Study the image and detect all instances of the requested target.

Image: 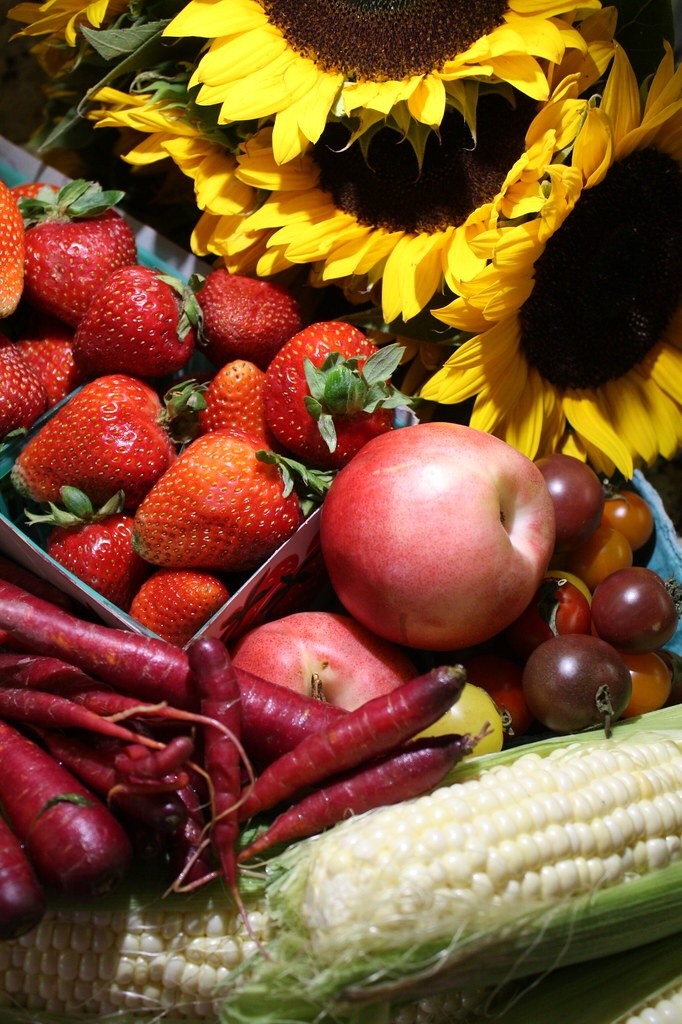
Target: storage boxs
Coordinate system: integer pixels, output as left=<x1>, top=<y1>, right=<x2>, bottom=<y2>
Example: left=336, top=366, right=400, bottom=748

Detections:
left=1, top=137, right=433, bottom=657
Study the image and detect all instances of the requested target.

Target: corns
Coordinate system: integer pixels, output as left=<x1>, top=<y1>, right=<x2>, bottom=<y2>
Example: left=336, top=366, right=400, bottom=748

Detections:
left=1, top=702, right=682, bottom=1024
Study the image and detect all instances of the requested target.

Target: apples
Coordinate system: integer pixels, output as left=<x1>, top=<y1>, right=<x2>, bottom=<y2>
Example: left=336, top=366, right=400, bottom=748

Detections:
left=230, top=419, right=553, bottom=714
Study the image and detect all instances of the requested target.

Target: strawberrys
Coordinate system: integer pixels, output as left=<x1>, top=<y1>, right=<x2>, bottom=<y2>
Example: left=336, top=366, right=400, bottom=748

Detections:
left=0, top=179, right=404, bottom=653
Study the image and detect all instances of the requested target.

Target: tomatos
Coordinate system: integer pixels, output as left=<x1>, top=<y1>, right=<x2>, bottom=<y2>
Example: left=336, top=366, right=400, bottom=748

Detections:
left=488, top=454, right=681, bottom=736
